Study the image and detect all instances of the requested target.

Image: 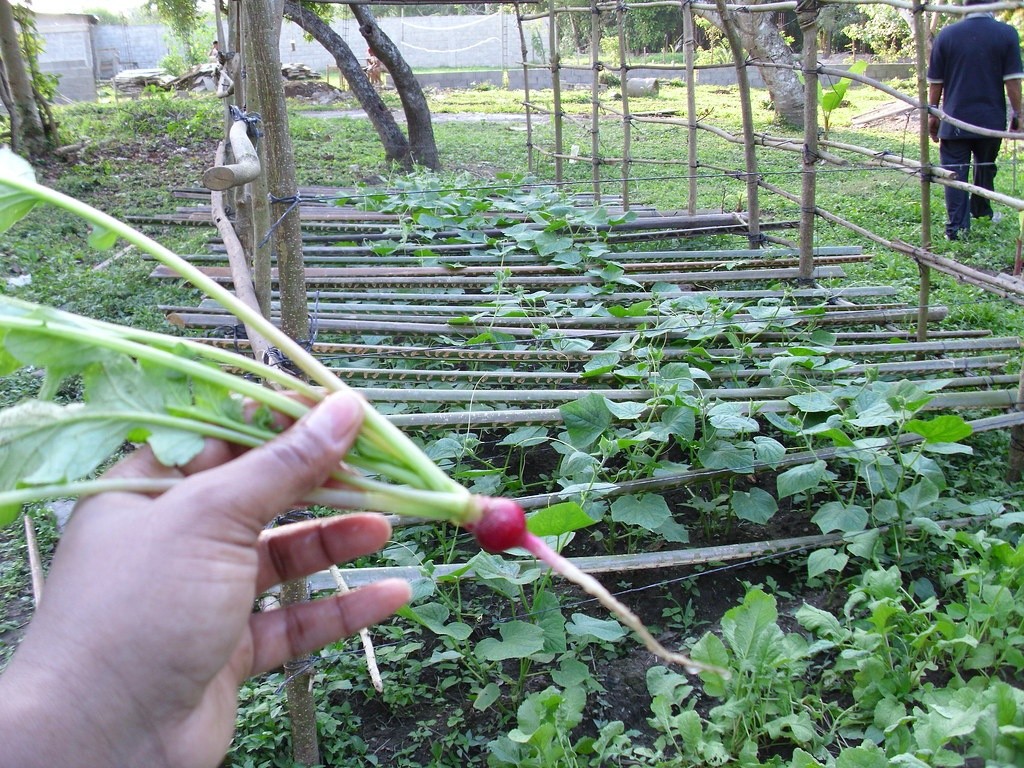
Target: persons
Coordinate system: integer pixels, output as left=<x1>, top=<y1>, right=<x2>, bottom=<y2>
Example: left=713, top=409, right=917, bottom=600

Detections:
left=366, top=48, right=383, bottom=82
left=923, top=0, right=1024, bottom=240
left=0, top=386, right=410, bottom=768
left=210, top=41, right=219, bottom=57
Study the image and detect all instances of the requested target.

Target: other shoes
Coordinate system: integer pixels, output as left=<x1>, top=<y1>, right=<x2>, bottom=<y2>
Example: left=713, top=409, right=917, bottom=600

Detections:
left=943, top=230, right=951, bottom=242
left=991, top=212, right=1005, bottom=222
